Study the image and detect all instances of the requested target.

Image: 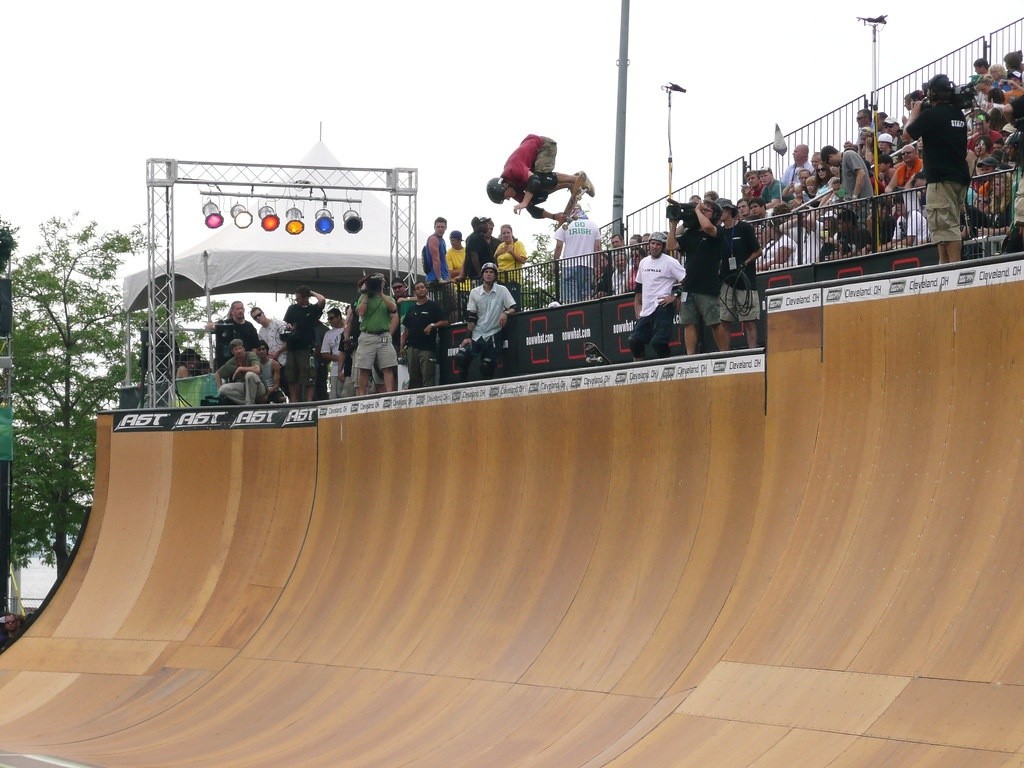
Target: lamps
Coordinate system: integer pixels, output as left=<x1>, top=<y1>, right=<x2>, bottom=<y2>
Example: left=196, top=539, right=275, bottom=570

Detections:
left=231, top=205, right=253, bottom=228
left=202, top=202, right=223, bottom=229
left=315, top=209, right=334, bottom=234
left=284, top=208, right=303, bottom=234
left=259, top=207, right=279, bottom=231
left=343, top=210, right=363, bottom=234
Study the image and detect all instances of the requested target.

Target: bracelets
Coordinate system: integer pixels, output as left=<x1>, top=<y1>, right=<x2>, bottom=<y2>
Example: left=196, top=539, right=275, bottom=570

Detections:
left=503, top=311, right=508, bottom=316
left=438, top=277, right=443, bottom=281
left=362, top=301, right=368, bottom=305
left=852, top=195, right=858, bottom=198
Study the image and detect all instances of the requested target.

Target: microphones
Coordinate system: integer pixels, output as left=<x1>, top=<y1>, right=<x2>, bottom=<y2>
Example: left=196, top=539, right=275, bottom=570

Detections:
left=667, top=198, right=678, bottom=204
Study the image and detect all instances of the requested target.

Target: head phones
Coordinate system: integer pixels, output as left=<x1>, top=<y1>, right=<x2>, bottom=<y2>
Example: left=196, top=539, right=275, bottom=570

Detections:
left=713, top=203, right=724, bottom=222
left=927, top=74, right=948, bottom=101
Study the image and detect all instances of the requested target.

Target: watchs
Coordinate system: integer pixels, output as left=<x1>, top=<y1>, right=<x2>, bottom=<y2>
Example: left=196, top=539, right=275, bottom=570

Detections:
left=673, top=292, right=678, bottom=298
left=430, top=323, right=435, bottom=327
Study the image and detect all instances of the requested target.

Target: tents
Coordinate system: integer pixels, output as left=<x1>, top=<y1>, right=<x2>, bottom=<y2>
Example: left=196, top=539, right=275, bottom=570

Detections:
left=121, top=142, right=431, bottom=388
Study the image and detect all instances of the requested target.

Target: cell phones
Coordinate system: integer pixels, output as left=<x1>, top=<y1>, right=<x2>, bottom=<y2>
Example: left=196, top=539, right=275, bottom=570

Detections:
left=1002, top=81, right=1007, bottom=85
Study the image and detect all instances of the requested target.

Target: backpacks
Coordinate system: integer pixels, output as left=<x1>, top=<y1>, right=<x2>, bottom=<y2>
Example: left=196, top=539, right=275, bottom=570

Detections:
left=421, top=235, right=441, bottom=276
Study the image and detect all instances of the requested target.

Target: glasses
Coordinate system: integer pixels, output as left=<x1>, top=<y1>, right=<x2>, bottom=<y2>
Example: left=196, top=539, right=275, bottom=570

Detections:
left=253, top=312, right=261, bottom=319
left=816, top=167, right=825, bottom=172
left=327, top=314, right=339, bottom=321
left=391, top=285, right=404, bottom=289
left=900, top=152, right=913, bottom=155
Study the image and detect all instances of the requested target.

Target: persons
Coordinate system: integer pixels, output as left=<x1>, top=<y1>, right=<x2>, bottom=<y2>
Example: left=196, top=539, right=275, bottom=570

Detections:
left=628, top=231, right=686, bottom=362
left=667, top=199, right=730, bottom=355
left=592, top=50, right=1024, bottom=299
left=554, top=203, right=603, bottom=305
left=903, top=74, right=970, bottom=265
left=451, top=261, right=517, bottom=380
left=175, top=215, right=527, bottom=405
left=714, top=198, right=762, bottom=350
left=487, top=135, right=595, bottom=223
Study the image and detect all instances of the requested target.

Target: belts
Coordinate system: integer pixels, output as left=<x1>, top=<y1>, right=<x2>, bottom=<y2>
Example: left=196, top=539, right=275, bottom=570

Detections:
left=363, top=330, right=389, bottom=335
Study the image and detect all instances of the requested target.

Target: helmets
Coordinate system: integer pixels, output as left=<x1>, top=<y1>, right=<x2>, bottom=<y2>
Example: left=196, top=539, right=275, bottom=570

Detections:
left=480, top=261, right=498, bottom=275
left=649, top=232, right=667, bottom=243
left=487, top=178, right=508, bottom=204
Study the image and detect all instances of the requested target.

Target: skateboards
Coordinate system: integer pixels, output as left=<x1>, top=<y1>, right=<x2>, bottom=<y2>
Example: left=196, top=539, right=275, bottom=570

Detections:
left=582, top=340, right=613, bottom=366
left=554, top=172, right=591, bottom=233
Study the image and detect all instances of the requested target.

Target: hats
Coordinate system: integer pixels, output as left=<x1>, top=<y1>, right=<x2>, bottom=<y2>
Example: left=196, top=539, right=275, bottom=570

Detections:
left=471, top=216, right=491, bottom=230
left=878, top=133, right=896, bottom=146
left=1007, top=70, right=1022, bottom=80
left=715, top=198, right=736, bottom=210
left=449, top=230, right=464, bottom=241
left=999, top=122, right=1016, bottom=133
left=976, top=157, right=999, bottom=168
left=859, top=126, right=872, bottom=135
left=228, top=338, right=244, bottom=349
left=820, top=211, right=837, bottom=218
left=754, top=166, right=773, bottom=174
